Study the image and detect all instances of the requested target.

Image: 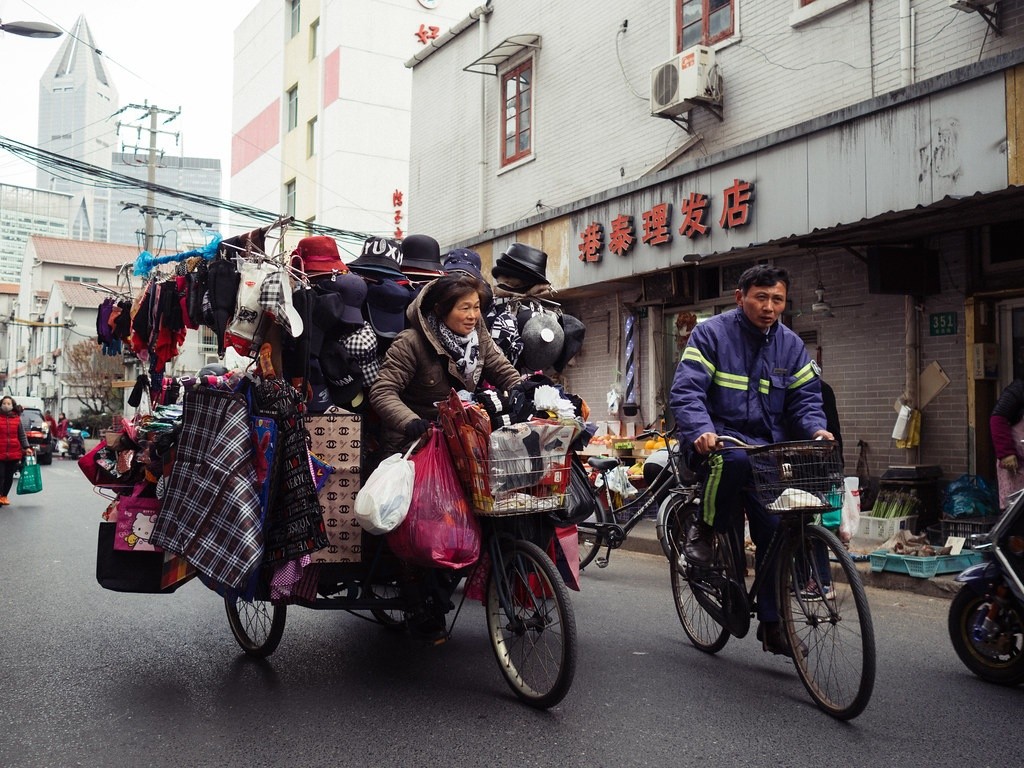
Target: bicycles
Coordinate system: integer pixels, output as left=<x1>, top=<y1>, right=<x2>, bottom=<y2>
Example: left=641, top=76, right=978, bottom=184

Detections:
left=670, top=436, right=879, bottom=721
left=573, top=424, right=724, bottom=582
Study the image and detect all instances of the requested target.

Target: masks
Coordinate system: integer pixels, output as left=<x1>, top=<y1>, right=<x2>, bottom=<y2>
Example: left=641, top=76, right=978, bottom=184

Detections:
left=1, top=404, right=13, bottom=412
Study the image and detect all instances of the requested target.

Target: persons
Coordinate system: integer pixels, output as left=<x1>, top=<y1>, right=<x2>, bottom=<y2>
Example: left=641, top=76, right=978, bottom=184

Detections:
left=670, top=265, right=834, bottom=658
left=990, top=346, right=1024, bottom=554
left=0, top=396, right=32, bottom=504
left=370, top=273, right=522, bottom=645
left=784, top=378, right=844, bottom=601
left=45, top=409, right=68, bottom=460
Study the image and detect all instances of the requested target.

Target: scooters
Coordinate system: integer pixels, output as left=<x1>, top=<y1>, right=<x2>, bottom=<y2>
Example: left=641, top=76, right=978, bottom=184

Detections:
left=66, top=421, right=89, bottom=460
left=945, top=488, right=1024, bottom=689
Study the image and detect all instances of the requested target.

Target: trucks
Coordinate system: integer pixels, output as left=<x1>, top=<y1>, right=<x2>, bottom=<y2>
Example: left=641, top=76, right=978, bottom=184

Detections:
left=11, top=395, right=46, bottom=415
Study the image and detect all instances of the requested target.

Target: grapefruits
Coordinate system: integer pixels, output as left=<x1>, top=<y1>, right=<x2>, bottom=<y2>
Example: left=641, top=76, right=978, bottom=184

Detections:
left=645, top=433, right=677, bottom=450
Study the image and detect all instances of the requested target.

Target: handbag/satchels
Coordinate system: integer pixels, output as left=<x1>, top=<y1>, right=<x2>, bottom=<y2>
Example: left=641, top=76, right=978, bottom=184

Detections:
left=386, top=428, right=481, bottom=570
left=821, top=482, right=842, bottom=528
left=489, top=420, right=575, bottom=495
left=837, top=476, right=861, bottom=542
left=17, top=455, right=43, bottom=495
left=353, top=437, right=421, bottom=535
left=113, top=478, right=165, bottom=553
left=547, top=451, right=596, bottom=526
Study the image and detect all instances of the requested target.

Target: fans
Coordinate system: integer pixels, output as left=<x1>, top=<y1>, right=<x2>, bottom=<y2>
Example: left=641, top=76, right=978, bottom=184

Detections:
left=784, top=250, right=866, bottom=319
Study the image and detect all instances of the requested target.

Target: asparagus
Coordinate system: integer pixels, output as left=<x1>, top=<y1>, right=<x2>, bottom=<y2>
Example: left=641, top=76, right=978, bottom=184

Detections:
left=627, top=461, right=644, bottom=475
left=870, top=488, right=921, bottom=519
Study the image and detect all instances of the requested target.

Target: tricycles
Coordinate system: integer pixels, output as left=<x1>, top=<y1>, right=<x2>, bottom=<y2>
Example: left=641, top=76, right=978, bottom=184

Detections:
left=176, top=380, right=580, bottom=711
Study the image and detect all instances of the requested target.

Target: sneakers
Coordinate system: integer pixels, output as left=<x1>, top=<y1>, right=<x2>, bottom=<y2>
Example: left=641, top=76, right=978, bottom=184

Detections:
left=787, top=578, right=813, bottom=596
left=799, top=580, right=836, bottom=602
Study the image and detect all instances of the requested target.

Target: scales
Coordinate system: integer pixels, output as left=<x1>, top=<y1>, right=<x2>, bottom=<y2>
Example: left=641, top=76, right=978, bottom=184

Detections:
left=879, top=464, right=944, bottom=480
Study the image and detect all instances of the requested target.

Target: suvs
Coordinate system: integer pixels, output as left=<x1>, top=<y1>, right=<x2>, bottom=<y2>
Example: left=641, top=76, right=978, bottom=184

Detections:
left=19, top=407, right=53, bottom=466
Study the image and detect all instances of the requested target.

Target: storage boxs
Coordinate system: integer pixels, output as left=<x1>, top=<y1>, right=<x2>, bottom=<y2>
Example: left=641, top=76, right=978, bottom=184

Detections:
left=855, top=511, right=918, bottom=541
left=871, top=550, right=986, bottom=580
left=938, top=511, right=998, bottom=546
left=613, top=498, right=632, bottom=524
left=632, top=489, right=659, bottom=518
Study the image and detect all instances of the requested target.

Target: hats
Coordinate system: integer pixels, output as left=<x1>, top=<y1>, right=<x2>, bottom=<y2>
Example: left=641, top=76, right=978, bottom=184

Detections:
left=258, top=232, right=586, bottom=412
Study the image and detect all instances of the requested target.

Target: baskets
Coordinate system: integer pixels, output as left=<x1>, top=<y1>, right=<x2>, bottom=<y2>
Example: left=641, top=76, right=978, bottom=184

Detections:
left=449, top=452, right=572, bottom=517
left=746, top=439, right=845, bottom=513
left=868, top=549, right=982, bottom=578
left=670, top=441, right=701, bottom=486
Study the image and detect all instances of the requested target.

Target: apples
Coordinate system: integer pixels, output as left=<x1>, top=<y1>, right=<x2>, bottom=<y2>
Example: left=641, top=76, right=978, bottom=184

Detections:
left=588, top=435, right=635, bottom=448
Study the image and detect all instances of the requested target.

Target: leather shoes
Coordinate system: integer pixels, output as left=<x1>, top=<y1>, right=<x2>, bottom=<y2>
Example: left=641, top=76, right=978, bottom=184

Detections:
left=683, top=519, right=713, bottom=567
left=0, top=496, right=9, bottom=505
left=756, top=621, right=808, bottom=658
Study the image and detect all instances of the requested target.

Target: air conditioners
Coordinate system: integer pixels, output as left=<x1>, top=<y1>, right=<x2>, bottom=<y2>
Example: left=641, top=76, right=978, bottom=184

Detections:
left=649, top=44, right=719, bottom=116
